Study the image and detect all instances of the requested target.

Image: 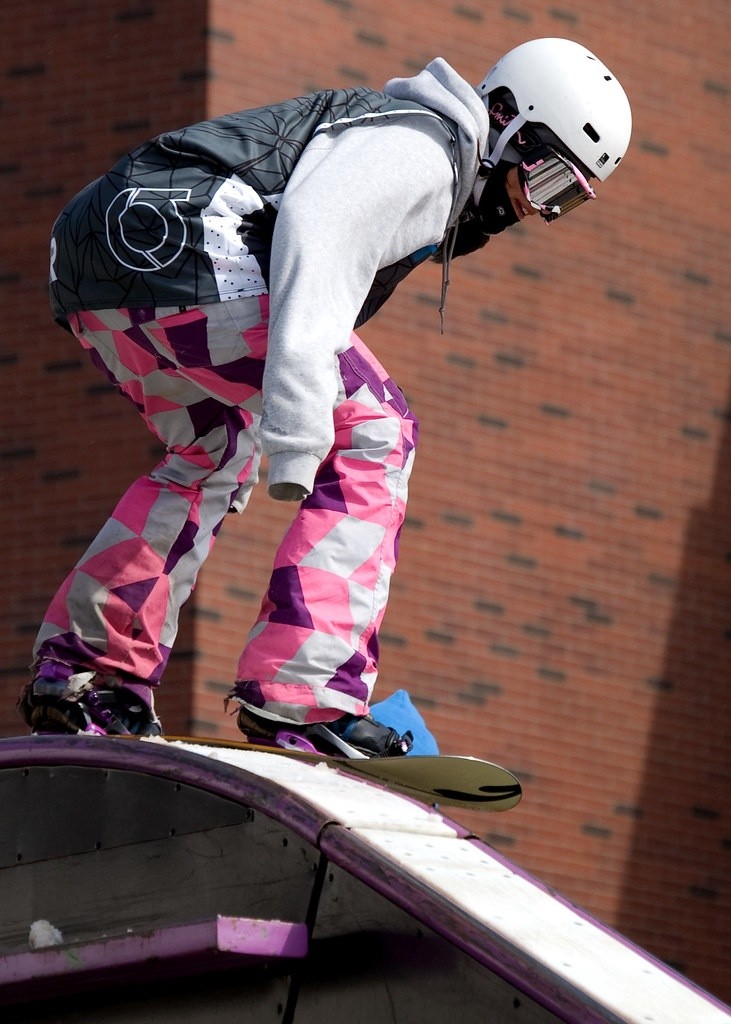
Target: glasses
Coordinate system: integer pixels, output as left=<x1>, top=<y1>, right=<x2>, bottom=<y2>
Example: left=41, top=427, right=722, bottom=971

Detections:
left=518, top=144, right=597, bottom=226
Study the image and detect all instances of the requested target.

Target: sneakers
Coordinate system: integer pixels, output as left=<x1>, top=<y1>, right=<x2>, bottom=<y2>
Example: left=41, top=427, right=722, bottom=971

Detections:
left=18, top=678, right=160, bottom=735
left=238, top=706, right=413, bottom=757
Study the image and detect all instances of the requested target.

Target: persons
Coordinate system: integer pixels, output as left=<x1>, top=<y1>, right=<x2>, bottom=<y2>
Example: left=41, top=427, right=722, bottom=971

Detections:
left=19, top=36, right=633, bottom=758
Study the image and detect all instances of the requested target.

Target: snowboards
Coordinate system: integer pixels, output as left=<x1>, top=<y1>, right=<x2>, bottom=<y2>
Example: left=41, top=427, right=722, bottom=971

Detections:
left=104, top=731, right=523, bottom=812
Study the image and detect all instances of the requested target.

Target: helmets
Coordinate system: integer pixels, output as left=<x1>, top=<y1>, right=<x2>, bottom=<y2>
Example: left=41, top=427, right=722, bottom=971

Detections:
left=477, top=37, right=633, bottom=182
